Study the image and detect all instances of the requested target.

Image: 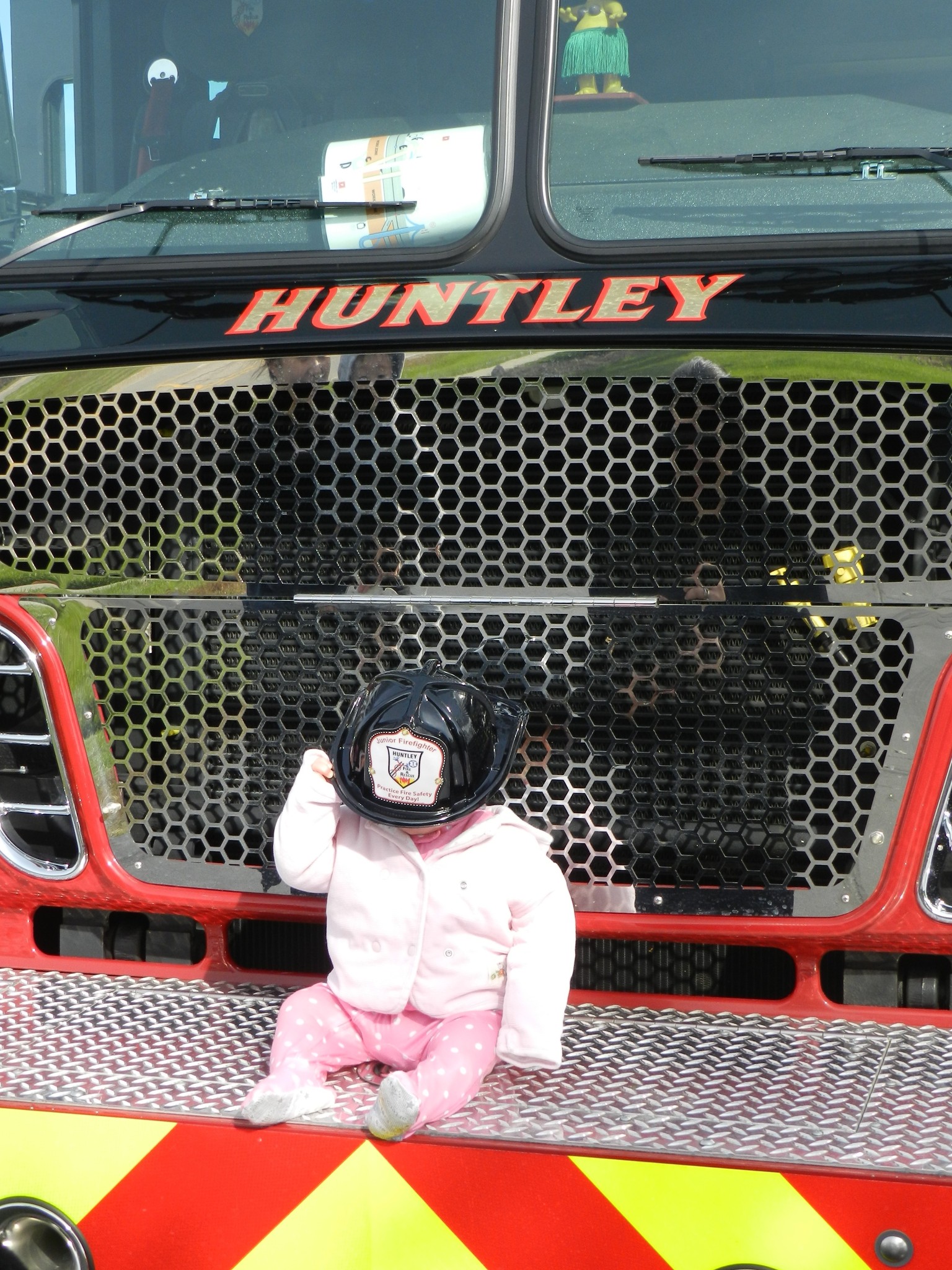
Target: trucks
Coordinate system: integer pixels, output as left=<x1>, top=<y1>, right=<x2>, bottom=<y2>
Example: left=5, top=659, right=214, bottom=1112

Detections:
left=0, top=1, right=952, bottom=1270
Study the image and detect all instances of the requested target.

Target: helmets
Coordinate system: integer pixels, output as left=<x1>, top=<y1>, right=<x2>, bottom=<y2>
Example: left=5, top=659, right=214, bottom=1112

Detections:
left=327, top=657, right=528, bottom=828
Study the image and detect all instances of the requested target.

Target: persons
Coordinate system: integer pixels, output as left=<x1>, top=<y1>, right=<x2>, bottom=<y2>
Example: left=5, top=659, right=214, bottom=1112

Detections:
left=239, top=659, right=576, bottom=1144
